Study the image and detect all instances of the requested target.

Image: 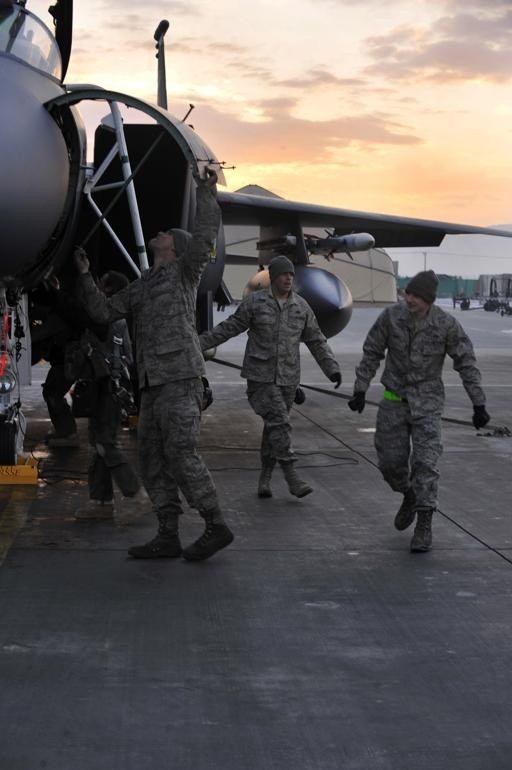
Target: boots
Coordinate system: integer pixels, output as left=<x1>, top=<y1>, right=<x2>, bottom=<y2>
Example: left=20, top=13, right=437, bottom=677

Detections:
left=258, top=468, right=272, bottom=497
left=411, top=506, right=433, bottom=551
left=280, top=460, right=313, bottom=498
left=395, top=487, right=416, bottom=531
left=183, top=507, right=233, bottom=560
left=128, top=520, right=181, bottom=558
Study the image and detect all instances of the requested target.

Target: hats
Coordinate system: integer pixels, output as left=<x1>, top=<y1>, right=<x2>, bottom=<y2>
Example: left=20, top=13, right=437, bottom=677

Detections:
left=405, top=271, right=440, bottom=305
left=171, top=228, right=192, bottom=259
left=268, top=256, right=295, bottom=280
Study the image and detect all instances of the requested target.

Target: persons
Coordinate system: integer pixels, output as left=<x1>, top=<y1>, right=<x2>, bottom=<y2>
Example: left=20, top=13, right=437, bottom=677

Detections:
left=30, top=265, right=83, bottom=449
left=70, top=271, right=140, bottom=521
left=197, top=255, right=344, bottom=497
left=71, top=164, right=233, bottom=564
left=347, top=269, right=492, bottom=553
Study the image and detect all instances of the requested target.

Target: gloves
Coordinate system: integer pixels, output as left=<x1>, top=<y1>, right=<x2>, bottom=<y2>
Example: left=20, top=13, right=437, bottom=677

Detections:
left=348, top=391, right=365, bottom=413
left=330, top=373, right=341, bottom=389
left=473, top=405, right=490, bottom=430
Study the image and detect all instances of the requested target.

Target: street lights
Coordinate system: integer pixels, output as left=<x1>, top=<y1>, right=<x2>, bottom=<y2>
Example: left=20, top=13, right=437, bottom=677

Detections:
left=421, top=251, right=428, bottom=273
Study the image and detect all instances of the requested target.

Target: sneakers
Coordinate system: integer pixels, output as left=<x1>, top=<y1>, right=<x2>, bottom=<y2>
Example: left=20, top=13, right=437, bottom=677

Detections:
left=49, top=433, right=78, bottom=447
left=75, top=500, right=116, bottom=518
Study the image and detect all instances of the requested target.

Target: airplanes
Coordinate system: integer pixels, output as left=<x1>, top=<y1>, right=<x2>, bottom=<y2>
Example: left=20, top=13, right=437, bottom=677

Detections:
left=0, top=0, right=512, bottom=464
